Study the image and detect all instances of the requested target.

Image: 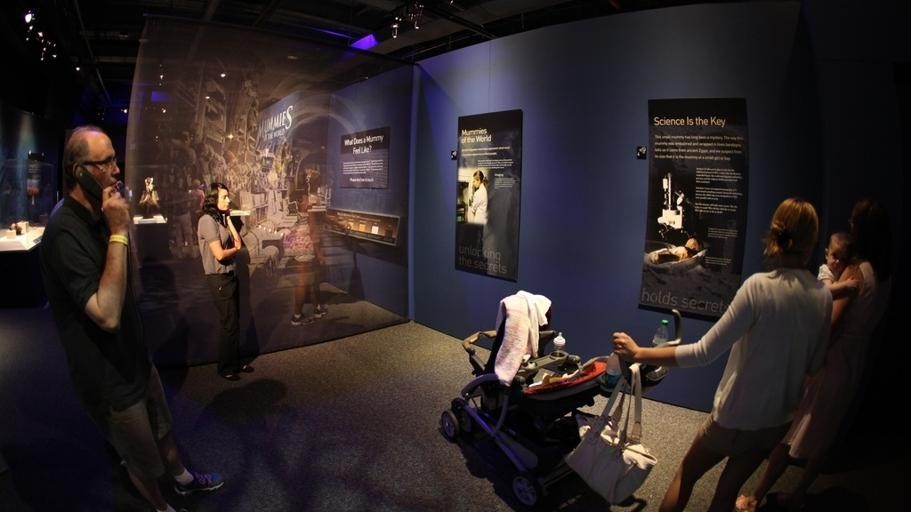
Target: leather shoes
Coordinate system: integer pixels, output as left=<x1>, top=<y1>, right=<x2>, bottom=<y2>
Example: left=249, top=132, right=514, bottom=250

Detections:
left=225, top=373, right=240, bottom=381
left=241, top=365, right=254, bottom=372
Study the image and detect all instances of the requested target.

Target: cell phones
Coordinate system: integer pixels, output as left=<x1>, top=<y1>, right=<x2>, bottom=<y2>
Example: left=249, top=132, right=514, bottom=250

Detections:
left=73, top=165, right=103, bottom=202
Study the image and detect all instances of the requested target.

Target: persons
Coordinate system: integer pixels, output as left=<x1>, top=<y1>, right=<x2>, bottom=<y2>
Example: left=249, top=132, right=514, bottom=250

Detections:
left=464, top=170, right=490, bottom=255
left=43, top=122, right=226, bottom=511
left=817, top=229, right=860, bottom=295
left=648, top=236, right=703, bottom=266
left=158, top=128, right=282, bottom=263
left=286, top=168, right=347, bottom=328
left=194, top=181, right=255, bottom=382
left=611, top=196, right=833, bottom=511
left=733, top=197, right=896, bottom=510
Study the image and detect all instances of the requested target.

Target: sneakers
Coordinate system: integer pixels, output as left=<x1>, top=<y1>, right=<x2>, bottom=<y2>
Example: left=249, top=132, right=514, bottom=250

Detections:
left=173, top=471, right=225, bottom=495
left=291, top=314, right=316, bottom=326
left=314, top=305, right=328, bottom=319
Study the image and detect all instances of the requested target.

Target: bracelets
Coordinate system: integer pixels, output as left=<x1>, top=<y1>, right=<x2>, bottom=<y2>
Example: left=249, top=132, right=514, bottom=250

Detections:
left=108, top=233, right=130, bottom=246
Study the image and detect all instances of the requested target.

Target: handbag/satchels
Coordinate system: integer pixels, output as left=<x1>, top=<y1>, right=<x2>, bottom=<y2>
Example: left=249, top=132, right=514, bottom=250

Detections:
left=565, top=414, right=659, bottom=505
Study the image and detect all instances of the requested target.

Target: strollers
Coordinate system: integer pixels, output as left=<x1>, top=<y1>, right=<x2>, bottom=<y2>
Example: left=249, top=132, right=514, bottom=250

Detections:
left=437, top=303, right=686, bottom=509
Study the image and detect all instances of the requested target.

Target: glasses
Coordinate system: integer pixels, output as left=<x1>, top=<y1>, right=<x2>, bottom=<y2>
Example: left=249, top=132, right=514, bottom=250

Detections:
left=82, top=157, right=117, bottom=170
